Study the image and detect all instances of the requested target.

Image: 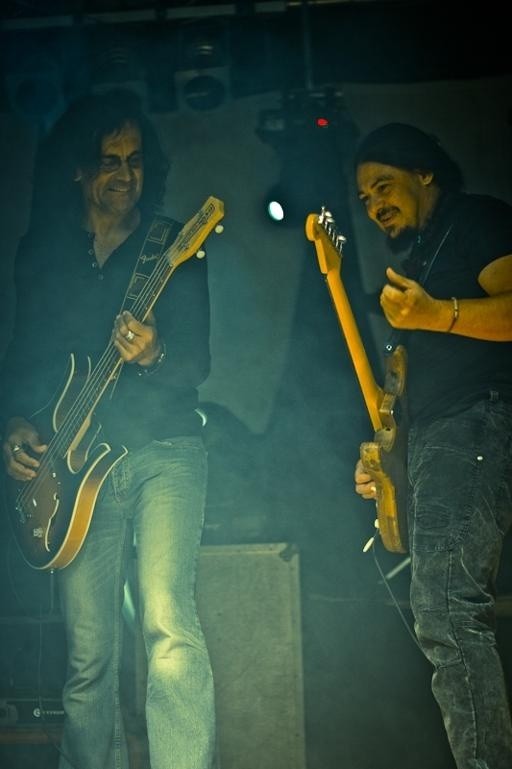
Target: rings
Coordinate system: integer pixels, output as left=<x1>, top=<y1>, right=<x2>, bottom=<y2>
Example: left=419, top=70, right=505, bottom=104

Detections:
left=12, top=444, right=25, bottom=457
left=125, top=330, right=135, bottom=341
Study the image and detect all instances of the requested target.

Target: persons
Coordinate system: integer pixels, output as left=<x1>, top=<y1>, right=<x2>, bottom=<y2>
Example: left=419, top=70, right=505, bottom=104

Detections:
left=353, top=123, right=512, bottom=769
left=0, top=95, right=218, bottom=769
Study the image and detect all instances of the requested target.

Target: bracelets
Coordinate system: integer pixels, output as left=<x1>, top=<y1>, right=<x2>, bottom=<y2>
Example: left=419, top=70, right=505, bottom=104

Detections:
left=135, top=343, right=165, bottom=377
left=444, top=297, right=460, bottom=334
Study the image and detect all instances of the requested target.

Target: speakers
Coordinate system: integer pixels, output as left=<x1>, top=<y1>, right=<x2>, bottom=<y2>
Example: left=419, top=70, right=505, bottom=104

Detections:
left=0, top=616, right=66, bottom=731
left=135, top=542, right=306, bottom=768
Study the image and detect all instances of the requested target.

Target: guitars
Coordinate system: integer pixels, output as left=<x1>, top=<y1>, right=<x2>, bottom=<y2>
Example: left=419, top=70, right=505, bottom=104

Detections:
left=1, top=196, right=225, bottom=570
left=306, top=206, right=409, bottom=554
